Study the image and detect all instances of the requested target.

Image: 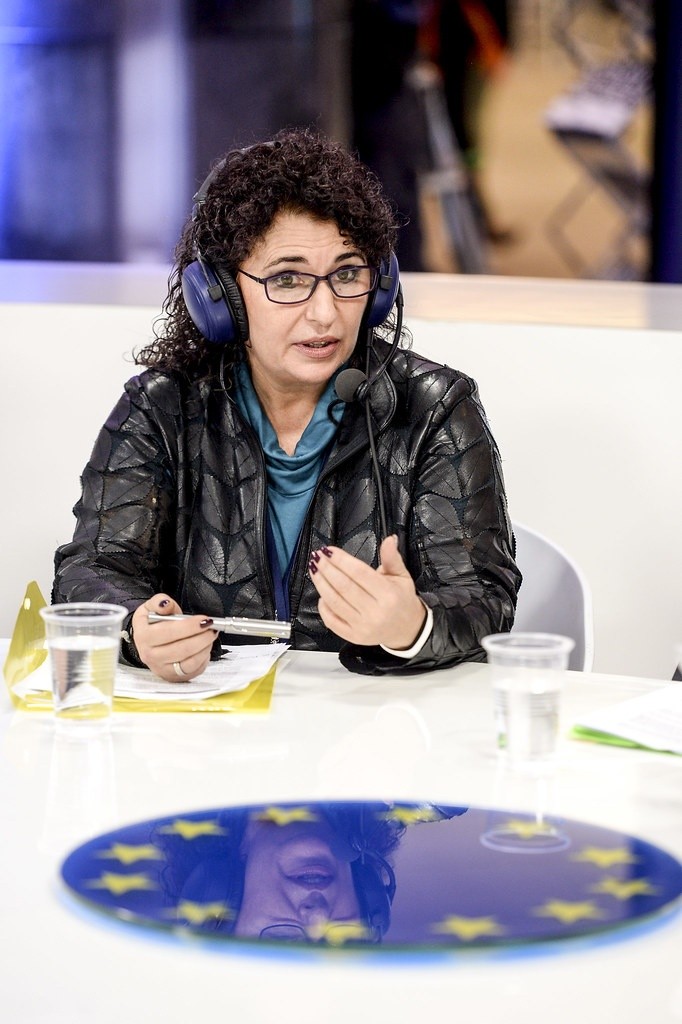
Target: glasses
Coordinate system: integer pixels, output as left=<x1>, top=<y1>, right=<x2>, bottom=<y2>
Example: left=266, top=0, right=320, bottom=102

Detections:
left=236, top=264, right=381, bottom=304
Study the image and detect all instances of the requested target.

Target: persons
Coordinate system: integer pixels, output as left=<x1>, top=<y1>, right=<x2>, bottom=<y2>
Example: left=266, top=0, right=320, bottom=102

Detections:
left=50, top=127, right=524, bottom=687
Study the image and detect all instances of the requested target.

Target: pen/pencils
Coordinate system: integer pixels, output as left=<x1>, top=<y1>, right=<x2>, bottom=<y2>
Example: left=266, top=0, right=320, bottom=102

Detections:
left=150, top=607, right=295, bottom=640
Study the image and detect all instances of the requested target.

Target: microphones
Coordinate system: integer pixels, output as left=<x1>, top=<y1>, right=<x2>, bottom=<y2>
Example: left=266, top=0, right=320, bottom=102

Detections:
left=335, top=285, right=404, bottom=401
left=328, top=830, right=397, bottom=901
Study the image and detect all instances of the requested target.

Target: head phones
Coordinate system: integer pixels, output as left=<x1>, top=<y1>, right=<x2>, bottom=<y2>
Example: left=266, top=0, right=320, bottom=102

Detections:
left=178, top=142, right=400, bottom=346
left=174, top=842, right=394, bottom=947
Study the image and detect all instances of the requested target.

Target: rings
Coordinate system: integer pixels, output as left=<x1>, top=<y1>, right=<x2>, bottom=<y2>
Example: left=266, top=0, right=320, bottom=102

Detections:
left=172, top=662, right=187, bottom=676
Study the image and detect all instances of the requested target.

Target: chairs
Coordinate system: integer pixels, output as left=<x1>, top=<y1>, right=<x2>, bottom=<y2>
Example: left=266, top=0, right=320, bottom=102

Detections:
left=505, top=524, right=597, bottom=670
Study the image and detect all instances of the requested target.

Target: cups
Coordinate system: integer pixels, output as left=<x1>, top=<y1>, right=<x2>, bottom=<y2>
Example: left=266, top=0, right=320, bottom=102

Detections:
left=40, top=604, right=128, bottom=724
left=481, top=632, right=574, bottom=758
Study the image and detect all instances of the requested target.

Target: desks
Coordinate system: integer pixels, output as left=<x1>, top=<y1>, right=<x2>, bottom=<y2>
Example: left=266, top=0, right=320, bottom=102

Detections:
left=0, top=635, right=681, bottom=1024
